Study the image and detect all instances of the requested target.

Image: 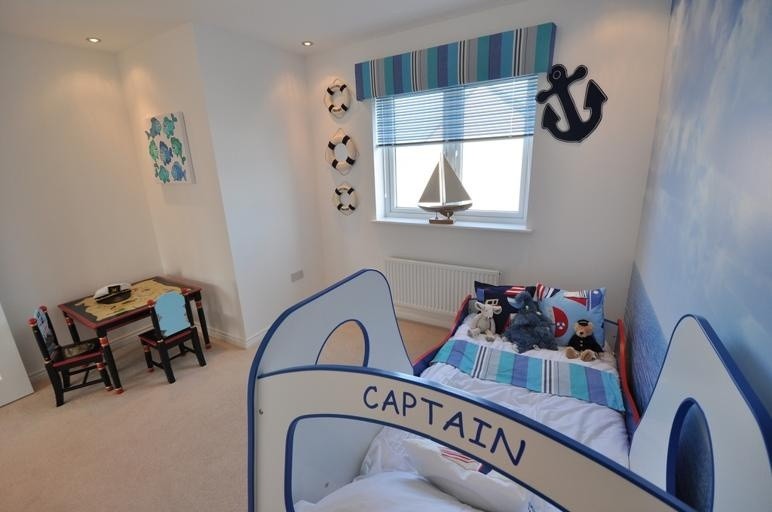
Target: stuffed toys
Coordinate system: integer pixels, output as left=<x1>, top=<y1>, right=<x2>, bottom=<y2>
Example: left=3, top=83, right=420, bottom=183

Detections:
left=566, top=319, right=603, bottom=361
left=502, top=290, right=560, bottom=352
left=468, top=301, right=502, bottom=341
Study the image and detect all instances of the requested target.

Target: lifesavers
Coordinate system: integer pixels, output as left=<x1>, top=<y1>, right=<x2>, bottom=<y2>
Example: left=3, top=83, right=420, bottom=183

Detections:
left=326, top=135, right=356, bottom=170
left=334, top=186, right=356, bottom=212
left=325, top=84, right=350, bottom=114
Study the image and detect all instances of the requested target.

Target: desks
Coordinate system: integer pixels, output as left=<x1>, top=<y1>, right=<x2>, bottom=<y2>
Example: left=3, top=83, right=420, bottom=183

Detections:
left=57, top=275, right=212, bottom=394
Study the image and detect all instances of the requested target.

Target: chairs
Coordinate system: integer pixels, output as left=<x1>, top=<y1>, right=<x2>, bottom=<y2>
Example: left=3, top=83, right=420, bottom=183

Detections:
left=136, top=288, right=206, bottom=384
left=27, top=305, right=113, bottom=407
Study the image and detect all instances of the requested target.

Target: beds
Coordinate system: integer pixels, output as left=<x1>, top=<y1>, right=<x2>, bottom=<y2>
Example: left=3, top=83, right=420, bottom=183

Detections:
left=247, top=268, right=772, bottom=512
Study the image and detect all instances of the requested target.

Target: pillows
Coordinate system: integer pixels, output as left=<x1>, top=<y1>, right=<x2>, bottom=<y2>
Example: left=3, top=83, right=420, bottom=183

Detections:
left=536, top=280, right=608, bottom=349
left=294, top=470, right=485, bottom=511
left=403, top=435, right=562, bottom=511
left=473, top=280, right=537, bottom=334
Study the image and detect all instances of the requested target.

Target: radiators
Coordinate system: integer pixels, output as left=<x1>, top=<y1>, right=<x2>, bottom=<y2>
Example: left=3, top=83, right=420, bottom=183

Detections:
left=383, top=257, right=501, bottom=317
